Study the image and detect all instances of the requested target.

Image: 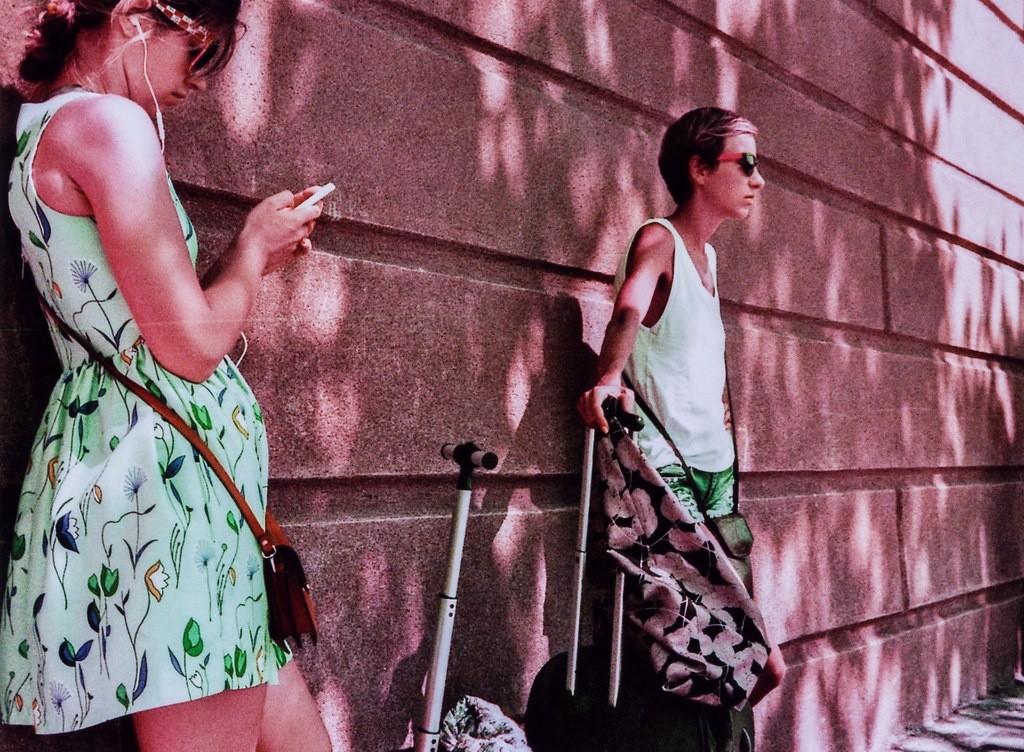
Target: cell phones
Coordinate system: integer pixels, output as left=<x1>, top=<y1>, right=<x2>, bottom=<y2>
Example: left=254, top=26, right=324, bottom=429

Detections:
left=293, top=183, right=336, bottom=212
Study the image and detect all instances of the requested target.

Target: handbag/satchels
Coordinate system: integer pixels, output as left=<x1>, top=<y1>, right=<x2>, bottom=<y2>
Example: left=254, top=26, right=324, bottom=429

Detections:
left=261, top=510, right=319, bottom=654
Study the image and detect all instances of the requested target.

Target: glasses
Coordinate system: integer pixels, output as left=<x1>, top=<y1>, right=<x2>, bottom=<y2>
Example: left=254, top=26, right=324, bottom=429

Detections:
left=150, top=0, right=220, bottom=76
left=716, top=150, right=759, bottom=177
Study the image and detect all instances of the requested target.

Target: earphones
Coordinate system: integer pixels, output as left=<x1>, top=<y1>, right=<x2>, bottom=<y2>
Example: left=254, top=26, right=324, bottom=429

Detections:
left=128, top=14, right=145, bottom=42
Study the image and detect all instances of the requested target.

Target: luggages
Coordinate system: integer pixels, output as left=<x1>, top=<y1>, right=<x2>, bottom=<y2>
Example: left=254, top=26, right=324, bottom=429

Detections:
left=525, top=401, right=758, bottom=752
left=418, top=437, right=530, bottom=752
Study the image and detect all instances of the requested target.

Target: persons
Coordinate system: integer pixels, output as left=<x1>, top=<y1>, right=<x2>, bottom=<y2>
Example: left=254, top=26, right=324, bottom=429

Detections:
left=579, top=106, right=786, bottom=705
left=0, top=0, right=334, bottom=752
